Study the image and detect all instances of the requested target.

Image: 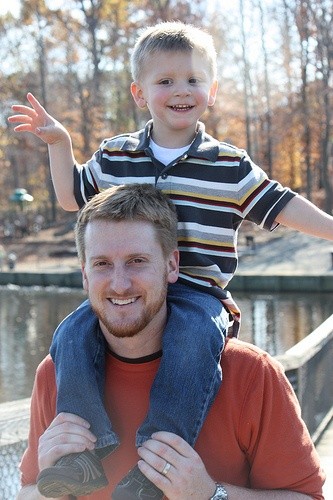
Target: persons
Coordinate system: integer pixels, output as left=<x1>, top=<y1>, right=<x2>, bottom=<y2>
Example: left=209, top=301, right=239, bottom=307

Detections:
left=17, top=185, right=326, bottom=500
left=7, top=22, right=332, bottom=500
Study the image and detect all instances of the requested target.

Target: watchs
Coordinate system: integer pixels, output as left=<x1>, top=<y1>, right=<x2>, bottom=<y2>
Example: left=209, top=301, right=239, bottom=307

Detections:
left=210, top=480, right=228, bottom=500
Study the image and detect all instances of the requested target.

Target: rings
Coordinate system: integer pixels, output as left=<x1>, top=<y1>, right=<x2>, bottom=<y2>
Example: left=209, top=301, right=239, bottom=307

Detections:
left=161, top=463, right=171, bottom=475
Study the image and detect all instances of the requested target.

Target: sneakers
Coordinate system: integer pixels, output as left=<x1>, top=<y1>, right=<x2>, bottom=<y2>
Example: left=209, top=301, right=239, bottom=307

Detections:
left=36, top=448, right=109, bottom=499
left=111, top=455, right=166, bottom=500
